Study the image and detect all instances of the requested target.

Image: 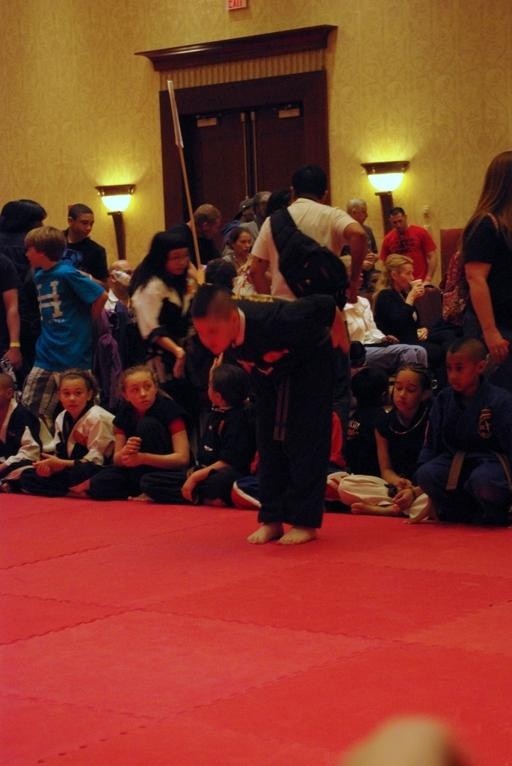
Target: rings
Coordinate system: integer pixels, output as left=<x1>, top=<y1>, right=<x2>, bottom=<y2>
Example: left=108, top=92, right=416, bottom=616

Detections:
left=494, top=350, right=499, bottom=353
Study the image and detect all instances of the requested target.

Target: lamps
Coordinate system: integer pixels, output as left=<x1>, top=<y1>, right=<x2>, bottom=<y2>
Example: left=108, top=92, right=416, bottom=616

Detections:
left=95, top=184, right=136, bottom=260
left=361, top=161, right=410, bottom=237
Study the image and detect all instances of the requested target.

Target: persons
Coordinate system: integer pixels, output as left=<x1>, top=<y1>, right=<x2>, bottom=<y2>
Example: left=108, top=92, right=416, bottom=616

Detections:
left=190, top=283, right=352, bottom=544
left=462, top=151, right=511, bottom=391
left=417, top=338, right=512, bottom=526
left=1, top=166, right=443, bottom=523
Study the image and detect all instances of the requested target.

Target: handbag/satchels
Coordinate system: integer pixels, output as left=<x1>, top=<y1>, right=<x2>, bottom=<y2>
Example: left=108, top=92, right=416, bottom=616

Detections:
left=442, top=212, right=499, bottom=326
left=277, top=232, right=347, bottom=311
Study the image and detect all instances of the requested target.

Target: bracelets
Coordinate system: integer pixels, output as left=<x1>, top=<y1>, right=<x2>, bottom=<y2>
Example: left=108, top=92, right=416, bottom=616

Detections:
left=410, top=488, right=417, bottom=498
left=9, top=343, right=20, bottom=347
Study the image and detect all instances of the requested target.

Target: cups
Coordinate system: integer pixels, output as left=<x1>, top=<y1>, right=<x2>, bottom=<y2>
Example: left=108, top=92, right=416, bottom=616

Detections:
left=408, top=278, right=423, bottom=296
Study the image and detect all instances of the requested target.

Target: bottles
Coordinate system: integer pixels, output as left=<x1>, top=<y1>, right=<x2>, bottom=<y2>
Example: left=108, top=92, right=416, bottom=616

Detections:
left=113, top=269, right=132, bottom=286
